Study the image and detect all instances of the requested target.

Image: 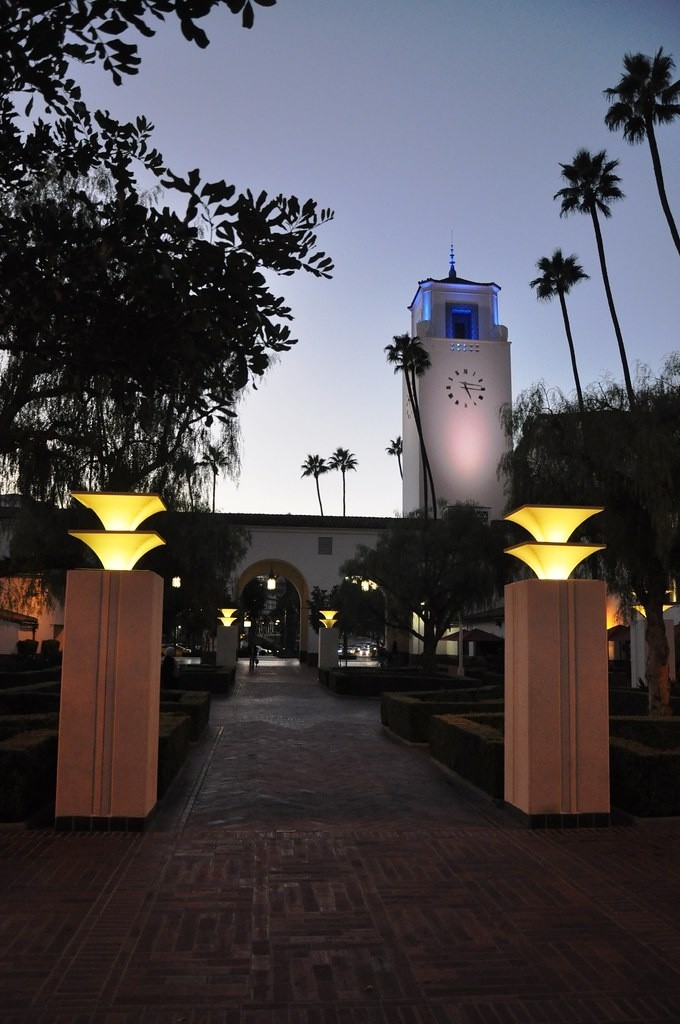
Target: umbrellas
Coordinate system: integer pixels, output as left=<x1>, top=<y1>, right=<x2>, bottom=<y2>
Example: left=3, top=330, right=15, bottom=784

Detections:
left=607, top=625, right=630, bottom=660
left=441, top=628, right=500, bottom=641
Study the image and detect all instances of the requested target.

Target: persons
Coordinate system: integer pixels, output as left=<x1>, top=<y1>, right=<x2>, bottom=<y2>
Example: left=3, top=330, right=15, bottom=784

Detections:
left=161, top=646, right=182, bottom=690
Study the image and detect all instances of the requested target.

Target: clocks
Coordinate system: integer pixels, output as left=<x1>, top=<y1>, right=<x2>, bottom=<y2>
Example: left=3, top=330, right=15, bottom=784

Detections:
left=446, top=369, right=486, bottom=407
